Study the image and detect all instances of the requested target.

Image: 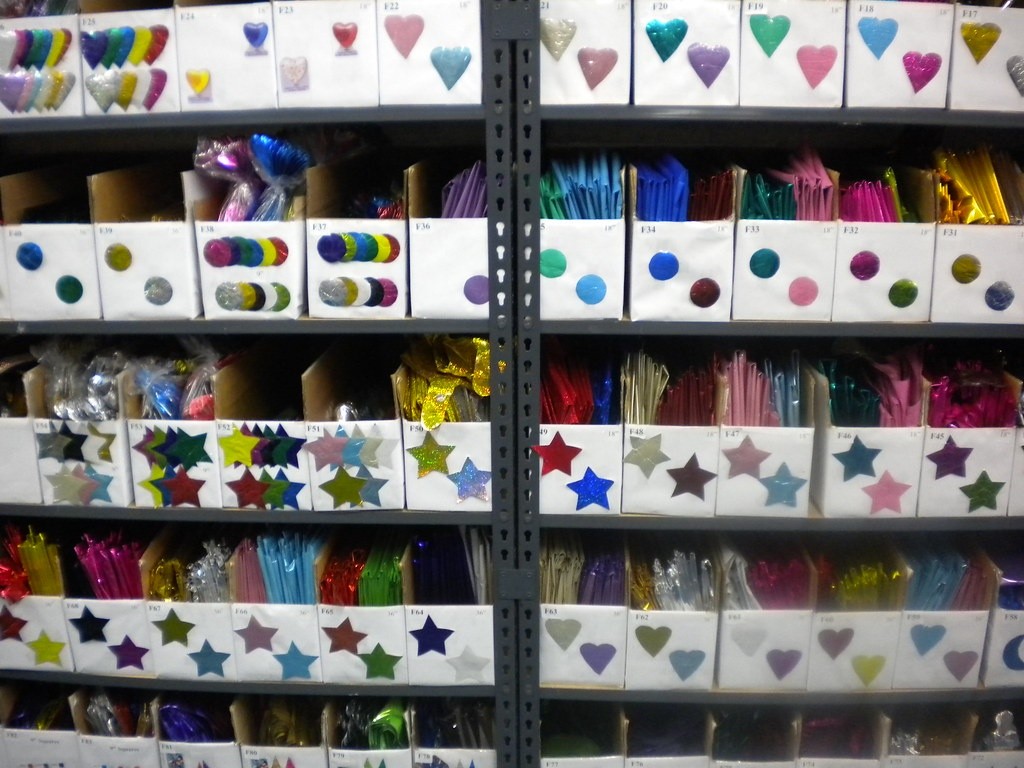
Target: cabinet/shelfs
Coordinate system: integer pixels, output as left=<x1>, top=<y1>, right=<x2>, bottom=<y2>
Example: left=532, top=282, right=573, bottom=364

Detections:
left=0, top=0, right=512, bottom=768
left=510, top=0, right=1024, bottom=768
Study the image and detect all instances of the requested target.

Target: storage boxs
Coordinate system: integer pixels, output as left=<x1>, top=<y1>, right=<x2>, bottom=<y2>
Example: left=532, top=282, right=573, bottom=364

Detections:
left=0, top=0, right=497, bottom=768
left=539, top=0, right=1024, bottom=768
